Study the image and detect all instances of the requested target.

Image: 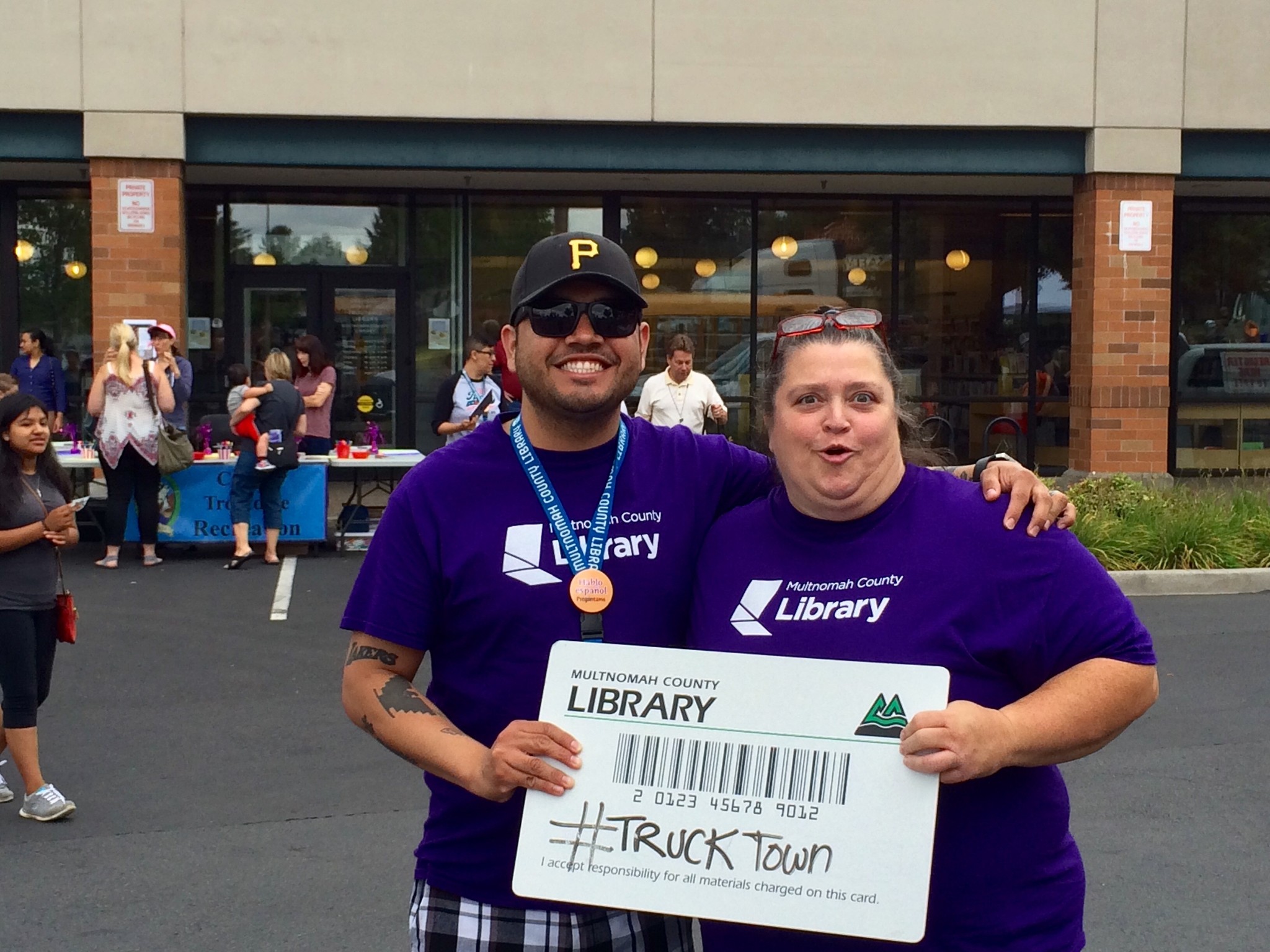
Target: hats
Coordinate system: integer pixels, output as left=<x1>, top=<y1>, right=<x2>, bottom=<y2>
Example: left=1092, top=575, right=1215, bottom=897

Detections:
left=510, top=230, right=649, bottom=325
left=146, top=324, right=176, bottom=339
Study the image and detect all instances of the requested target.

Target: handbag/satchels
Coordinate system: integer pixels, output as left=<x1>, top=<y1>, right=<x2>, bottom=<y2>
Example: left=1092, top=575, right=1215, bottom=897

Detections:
left=157, top=424, right=193, bottom=474
left=55, top=592, right=77, bottom=644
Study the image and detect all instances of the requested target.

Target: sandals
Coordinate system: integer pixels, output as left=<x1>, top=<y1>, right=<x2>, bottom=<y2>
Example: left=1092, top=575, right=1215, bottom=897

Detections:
left=96, top=556, right=118, bottom=569
left=143, top=554, right=163, bottom=567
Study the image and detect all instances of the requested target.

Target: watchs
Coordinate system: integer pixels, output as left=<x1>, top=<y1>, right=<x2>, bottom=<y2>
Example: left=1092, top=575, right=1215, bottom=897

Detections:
left=972, top=453, right=1022, bottom=483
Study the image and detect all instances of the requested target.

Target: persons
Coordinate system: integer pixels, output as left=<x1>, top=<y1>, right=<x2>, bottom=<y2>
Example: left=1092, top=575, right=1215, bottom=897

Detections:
left=340, top=231, right=1076, bottom=952
left=686, top=305, right=1159, bottom=952
left=0, top=392, right=80, bottom=819
left=87, top=323, right=176, bottom=568
left=1024, top=329, right=1069, bottom=447
left=292, top=334, right=337, bottom=455
left=147, top=323, right=193, bottom=428
left=224, top=351, right=307, bottom=570
left=430, top=334, right=504, bottom=445
left=226, top=364, right=276, bottom=470
left=0, top=373, right=19, bottom=399
left=491, top=340, right=523, bottom=412
left=635, top=334, right=728, bottom=436
left=9, top=328, right=67, bottom=441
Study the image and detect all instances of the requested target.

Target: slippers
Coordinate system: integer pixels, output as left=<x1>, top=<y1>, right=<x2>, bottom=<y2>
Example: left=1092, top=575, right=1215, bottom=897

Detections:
left=261, top=556, right=281, bottom=565
left=224, top=550, right=255, bottom=569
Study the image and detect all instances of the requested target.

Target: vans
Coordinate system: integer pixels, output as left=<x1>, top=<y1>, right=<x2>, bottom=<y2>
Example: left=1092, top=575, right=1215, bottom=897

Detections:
left=1175, top=331, right=1270, bottom=402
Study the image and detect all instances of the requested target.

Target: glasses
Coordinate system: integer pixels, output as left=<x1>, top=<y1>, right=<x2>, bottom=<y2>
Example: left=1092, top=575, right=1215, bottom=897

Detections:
left=474, top=349, right=494, bottom=357
left=514, top=293, right=640, bottom=337
left=772, top=308, right=886, bottom=361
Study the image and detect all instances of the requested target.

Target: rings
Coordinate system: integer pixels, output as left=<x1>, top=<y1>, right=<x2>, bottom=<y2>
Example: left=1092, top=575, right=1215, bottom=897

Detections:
left=1049, top=490, right=1059, bottom=496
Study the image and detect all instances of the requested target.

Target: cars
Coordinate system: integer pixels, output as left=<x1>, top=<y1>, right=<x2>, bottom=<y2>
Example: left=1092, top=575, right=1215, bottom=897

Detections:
left=628, top=331, right=779, bottom=403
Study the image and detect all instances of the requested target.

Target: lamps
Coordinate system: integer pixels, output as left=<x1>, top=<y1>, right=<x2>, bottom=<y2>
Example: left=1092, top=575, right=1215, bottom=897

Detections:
left=771, top=216, right=799, bottom=260
left=642, top=273, right=660, bottom=290
left=253, top=253, right=276, bottom=265
left=945, top=235, right=970, bottom=271
left=635, top=245, right=658, bottom=268
left=345, top=243, right=368, bottom=265
left=17, top=239, right=35, bottom=261
left=849, top=260, right=866, bottom=285
left=696, top=258, right=717, bottom=277
left=65, top=260, right=87, bottom=279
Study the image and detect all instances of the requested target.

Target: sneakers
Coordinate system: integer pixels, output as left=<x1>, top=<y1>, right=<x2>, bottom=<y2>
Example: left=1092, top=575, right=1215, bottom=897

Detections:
left=254, top=459, right=276, bottom=471
left=18, top=782, right=76, bottom=821
left=0, top=760, right=13, bottom=803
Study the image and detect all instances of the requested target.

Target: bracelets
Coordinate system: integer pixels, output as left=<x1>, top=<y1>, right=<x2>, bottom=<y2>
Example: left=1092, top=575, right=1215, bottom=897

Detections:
left=41, top=520, right=50, bottom=531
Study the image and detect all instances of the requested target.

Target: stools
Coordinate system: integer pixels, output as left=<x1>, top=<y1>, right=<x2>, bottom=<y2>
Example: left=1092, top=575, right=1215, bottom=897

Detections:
left=989, top=412, right=1028, bottom=462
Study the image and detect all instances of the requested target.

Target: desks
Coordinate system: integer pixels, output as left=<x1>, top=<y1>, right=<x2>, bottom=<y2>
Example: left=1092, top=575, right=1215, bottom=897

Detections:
left=50, top=448, right=426, bottom=555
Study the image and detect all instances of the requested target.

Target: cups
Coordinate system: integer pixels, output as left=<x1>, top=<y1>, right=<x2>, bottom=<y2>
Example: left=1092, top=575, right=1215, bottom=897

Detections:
left=218, top=449, right=231, bottom=460
left=81, top=444, right=95, bottom=459
left=336, top=445, right=350, bottom=458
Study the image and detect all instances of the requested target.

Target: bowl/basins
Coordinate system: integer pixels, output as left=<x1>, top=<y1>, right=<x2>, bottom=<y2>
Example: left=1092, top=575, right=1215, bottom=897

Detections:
left=351, top=452, right=370, bottom=459
left=194, top=452, right=206, bottom=460
